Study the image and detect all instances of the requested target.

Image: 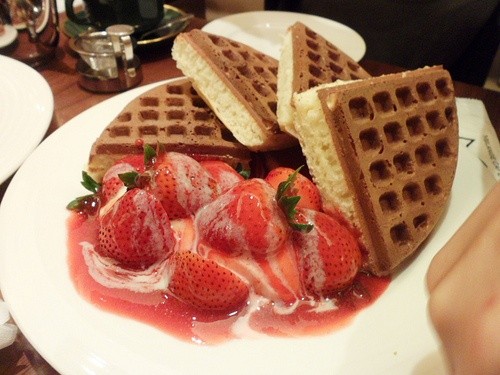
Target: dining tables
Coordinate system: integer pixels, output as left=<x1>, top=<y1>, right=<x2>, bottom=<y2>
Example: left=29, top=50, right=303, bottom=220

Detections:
left=0, top=0, right=500, bottom=375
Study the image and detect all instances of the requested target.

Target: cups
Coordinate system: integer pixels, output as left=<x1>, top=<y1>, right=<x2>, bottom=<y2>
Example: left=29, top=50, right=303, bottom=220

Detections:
left=68, top=22, right=143, bottom=92
left=65, top=0, right=164, bottom=34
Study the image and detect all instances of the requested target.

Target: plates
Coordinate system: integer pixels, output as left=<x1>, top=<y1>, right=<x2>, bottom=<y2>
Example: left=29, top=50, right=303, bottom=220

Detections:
left=0, top=53, right=54, bottom=185
left=202, top=9, right=367, bottom=67
left=0, top=77, right=461, bottom=375
left=61, top=4, right=192, bottom=54
left=0, top=23, right=17, bottom=48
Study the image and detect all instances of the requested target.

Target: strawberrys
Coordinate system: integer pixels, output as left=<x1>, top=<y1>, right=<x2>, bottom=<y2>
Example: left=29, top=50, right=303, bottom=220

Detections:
left=67, top=146, right=361, bottom=311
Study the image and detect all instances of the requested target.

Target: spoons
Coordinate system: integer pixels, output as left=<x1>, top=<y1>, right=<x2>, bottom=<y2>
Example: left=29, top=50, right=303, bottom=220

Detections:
left=131, top=15, right=194, bottom=40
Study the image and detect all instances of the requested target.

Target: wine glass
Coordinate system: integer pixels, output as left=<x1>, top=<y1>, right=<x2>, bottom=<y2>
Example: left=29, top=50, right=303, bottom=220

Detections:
left=0, top=0, right=59, bottom=67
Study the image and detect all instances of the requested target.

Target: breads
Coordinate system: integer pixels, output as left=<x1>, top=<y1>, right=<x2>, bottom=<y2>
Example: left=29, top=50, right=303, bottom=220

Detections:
left=171, top=28, right=299, bottom=153
left=276, top=21, right=375, bottom=141
left=85, top=79, right=253, bottom=180
left=294, top=64, right=458, bottom=278
